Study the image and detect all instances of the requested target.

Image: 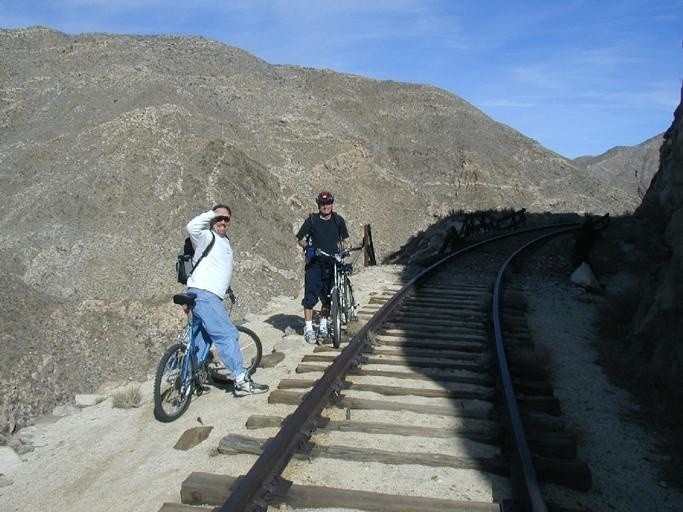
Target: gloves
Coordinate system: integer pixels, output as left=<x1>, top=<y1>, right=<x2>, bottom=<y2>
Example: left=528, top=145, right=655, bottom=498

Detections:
left=304, top=245, right=315, bottom=261
left=343, top=263, right=353, bottom=274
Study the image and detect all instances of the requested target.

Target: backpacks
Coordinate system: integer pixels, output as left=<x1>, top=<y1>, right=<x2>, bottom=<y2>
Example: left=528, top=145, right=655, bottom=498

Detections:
left=176, top=231, right=216, bottom=285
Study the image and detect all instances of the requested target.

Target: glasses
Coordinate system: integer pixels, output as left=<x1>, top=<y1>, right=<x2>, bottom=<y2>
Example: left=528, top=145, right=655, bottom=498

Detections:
left=215, top=217, right=230, bottom=222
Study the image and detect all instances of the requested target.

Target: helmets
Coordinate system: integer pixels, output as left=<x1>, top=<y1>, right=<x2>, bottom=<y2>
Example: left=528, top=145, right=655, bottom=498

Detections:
left=315, top=192, right=334, bottom=205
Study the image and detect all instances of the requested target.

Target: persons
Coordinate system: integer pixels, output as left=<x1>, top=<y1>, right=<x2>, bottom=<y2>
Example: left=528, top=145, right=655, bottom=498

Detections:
left=296, top=191, right=350, bottom=345
left=178, top=204, right=272, bottom=397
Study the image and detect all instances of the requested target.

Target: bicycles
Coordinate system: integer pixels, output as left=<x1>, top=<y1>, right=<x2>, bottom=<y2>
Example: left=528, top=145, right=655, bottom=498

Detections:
left=315, top=247, right=362, bottom=348
left=154, top=285, right=262, bottom=422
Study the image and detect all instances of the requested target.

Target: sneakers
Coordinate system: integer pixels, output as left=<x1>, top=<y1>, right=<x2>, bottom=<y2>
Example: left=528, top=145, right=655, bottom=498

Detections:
left=304, top=326, right=328, bottom=344
left=199, top=386, right=211, bottom=394
left=235, top=373, right=269, bottom=397
